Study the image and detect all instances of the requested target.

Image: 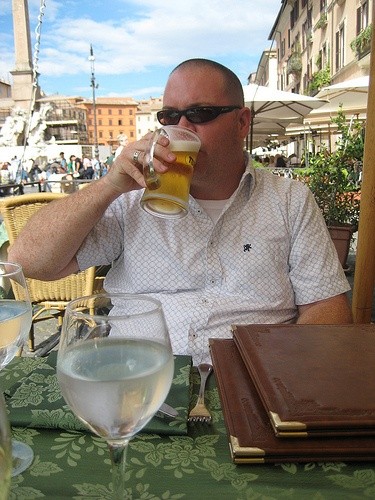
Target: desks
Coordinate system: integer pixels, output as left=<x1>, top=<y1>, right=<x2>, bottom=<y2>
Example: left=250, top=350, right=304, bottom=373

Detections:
left=0, top=357, right=374, bottom=500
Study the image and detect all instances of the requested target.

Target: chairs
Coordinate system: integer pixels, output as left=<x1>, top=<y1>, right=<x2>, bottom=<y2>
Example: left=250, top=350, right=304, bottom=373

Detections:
left=1, top=192, right=111, bottom=352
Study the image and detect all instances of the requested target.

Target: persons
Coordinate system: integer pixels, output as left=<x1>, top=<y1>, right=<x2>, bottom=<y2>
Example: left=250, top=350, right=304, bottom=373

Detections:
left=6, top=58, right=355, bottom=367
left=253, top=153, right=301, bottom=168
left=0, top=150, right=117, bottom=185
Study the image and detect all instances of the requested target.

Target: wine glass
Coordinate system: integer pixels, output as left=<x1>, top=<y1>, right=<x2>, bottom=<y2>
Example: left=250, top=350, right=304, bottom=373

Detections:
left=56, top=293, right=175, bottom=500
left=0, top=260, right=34, bottom=476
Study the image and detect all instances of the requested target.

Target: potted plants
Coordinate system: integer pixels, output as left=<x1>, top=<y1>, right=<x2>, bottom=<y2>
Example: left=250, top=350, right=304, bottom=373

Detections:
left=294, top=100, right=366, bottom=268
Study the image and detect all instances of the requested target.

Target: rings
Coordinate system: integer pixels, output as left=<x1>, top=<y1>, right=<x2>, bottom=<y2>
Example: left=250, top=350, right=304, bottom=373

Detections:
left=133, top=150, right=142, bottom=161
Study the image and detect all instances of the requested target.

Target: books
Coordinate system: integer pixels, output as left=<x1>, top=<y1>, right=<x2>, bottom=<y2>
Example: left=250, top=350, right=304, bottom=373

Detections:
left=207, top=322, right=375, bottom=464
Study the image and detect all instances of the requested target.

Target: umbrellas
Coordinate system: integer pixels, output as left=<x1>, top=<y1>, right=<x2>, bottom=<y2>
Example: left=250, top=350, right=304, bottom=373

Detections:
left=241, top=75, right=370, bottom=155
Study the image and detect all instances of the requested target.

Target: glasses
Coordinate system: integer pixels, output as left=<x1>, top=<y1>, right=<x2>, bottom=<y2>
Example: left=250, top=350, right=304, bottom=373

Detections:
left=157, top=105, right=245, bottom=127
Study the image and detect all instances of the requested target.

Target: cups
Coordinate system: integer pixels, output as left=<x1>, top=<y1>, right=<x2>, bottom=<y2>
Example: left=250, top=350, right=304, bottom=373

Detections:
left=0, top=390, right=13, bottom=500
left=139, top=124, right=203, bottom=220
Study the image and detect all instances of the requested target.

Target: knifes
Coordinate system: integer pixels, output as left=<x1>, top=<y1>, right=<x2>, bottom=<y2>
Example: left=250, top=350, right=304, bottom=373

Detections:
left=158, top=402, right=178, bottom=418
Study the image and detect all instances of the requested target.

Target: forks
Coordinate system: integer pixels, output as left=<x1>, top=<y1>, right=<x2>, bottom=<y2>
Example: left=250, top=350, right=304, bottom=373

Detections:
left=186, top=362, right=213, bottom=424
